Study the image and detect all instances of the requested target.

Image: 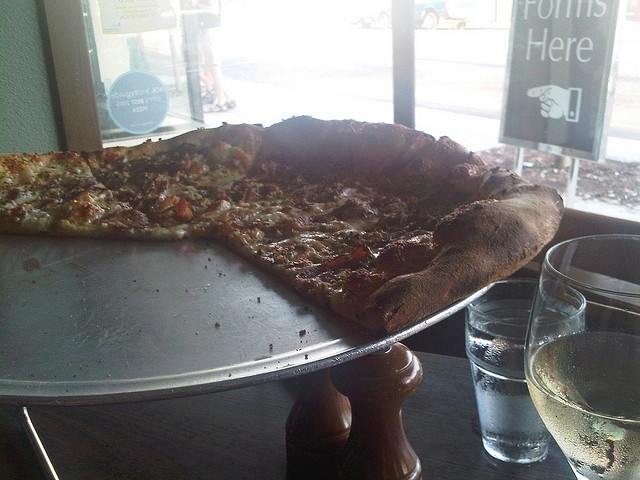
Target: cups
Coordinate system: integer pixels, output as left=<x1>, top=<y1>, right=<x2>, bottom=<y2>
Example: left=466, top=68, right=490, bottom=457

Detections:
left=463, top=278, right=584, bottom=462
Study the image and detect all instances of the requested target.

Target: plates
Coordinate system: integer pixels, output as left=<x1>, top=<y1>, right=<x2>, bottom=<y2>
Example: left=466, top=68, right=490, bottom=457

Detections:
left=2, top=173, right=558, bottom=406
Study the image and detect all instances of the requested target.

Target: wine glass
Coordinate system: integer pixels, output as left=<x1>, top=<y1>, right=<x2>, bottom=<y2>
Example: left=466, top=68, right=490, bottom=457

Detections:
left=524, top=234, right=640, bottom=479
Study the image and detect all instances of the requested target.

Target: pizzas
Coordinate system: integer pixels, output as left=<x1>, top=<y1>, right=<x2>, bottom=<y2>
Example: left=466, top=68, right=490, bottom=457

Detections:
left=0, top=113, right=565, bottom=334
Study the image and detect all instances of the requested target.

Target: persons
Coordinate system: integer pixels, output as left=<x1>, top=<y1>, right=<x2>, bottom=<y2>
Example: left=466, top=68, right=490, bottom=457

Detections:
left=527, top=85, right=581, bottom=123
